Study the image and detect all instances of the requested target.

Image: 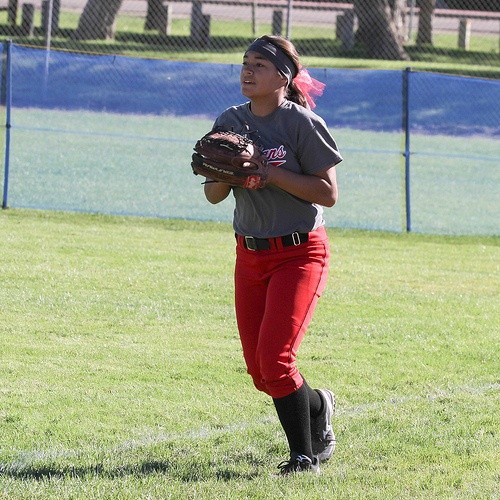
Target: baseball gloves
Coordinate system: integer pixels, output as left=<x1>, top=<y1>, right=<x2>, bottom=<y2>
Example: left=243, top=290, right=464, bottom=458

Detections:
left=191, top=121, right=269, bottom=192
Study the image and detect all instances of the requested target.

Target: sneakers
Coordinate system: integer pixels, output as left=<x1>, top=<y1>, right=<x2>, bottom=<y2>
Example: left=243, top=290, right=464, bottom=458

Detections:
left=312, top=388, right=336, bottom=461
left=273, top=452, right=320, bottom=478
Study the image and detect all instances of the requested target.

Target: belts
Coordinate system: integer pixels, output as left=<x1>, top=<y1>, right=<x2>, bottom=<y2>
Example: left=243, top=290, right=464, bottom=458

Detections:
left=234, top=232, right=308, bottom=251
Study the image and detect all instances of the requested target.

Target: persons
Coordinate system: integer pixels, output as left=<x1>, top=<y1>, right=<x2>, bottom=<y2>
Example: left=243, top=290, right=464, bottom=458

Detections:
left=190, top=34, right=344, bottom=481
